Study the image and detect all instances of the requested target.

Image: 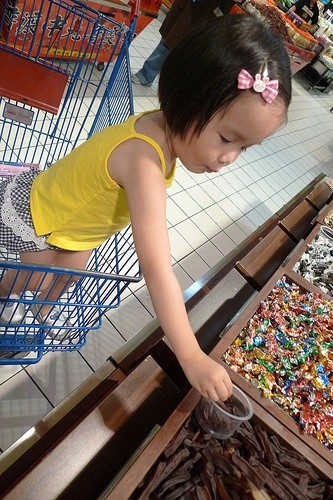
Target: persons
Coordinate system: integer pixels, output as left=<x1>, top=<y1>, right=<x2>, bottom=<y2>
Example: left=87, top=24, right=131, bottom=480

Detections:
left=131, top=0, right=237, bottom=87
left=0, top=14, right=292, bottom=404
left=285, top=0, right=321, bottom=26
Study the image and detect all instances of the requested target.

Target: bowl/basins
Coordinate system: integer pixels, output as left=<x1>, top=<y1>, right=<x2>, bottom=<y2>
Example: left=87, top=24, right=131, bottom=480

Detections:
left=195, top=385, right=253, bottom=440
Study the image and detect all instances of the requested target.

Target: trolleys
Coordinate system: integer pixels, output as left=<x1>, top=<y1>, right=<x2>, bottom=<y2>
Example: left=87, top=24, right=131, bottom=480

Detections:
left=0, top=0, right=145, bottom=366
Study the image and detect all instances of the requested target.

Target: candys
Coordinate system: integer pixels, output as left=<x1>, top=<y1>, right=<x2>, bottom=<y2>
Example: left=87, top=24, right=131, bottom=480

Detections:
left=220, top=209, right=333, bottom=451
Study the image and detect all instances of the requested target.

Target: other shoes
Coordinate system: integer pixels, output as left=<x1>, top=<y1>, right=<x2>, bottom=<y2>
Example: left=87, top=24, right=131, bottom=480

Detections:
left=131, top=74, right=151, bottom=87
left=31, top=293, right=71, bottom=340
left=0, top=293, right=26, bottom=327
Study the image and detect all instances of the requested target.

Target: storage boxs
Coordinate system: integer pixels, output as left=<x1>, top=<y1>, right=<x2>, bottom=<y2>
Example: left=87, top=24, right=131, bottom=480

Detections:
left=98, top=196, right=332, bottom=500
